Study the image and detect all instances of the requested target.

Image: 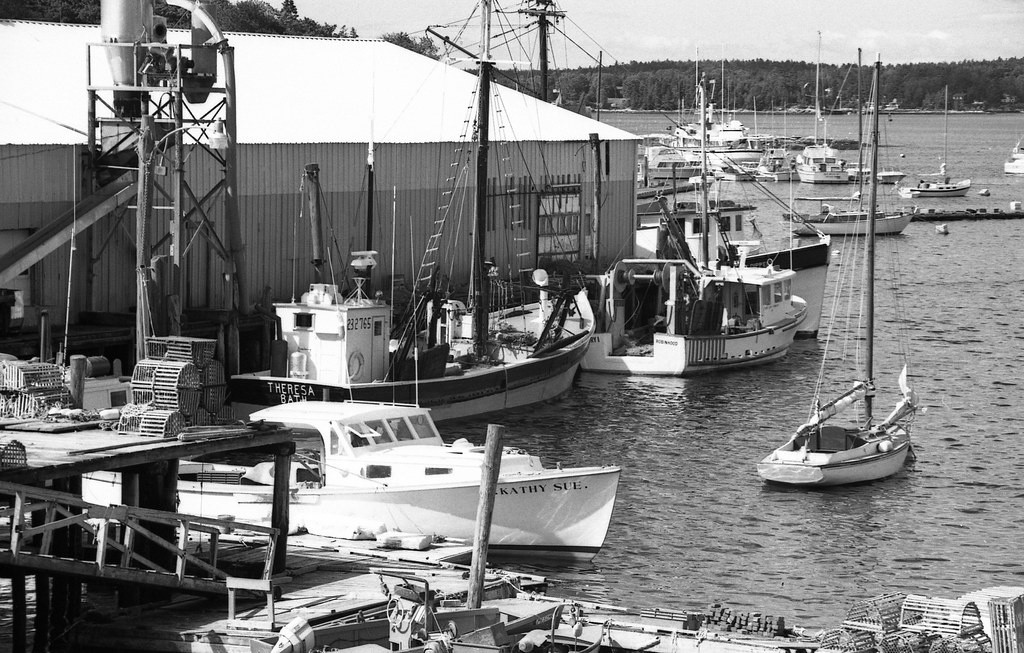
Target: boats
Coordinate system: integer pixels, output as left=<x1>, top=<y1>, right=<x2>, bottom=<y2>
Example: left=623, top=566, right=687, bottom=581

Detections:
left=79, top=396, right=623, bottom=563
left=577, top=42, right=809, bottom=380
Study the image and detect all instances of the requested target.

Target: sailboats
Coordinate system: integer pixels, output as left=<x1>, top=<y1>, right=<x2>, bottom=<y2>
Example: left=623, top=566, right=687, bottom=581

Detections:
left=226, top=1, right=600, bottom=429
left=755, top=53, right=927, bottom=488
left=636, top=28, right=1024, bottom=342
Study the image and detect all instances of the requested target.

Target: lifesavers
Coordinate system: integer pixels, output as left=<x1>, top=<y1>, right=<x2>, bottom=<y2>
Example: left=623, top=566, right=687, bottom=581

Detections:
left=348, top=351, right=364, bottom=381
